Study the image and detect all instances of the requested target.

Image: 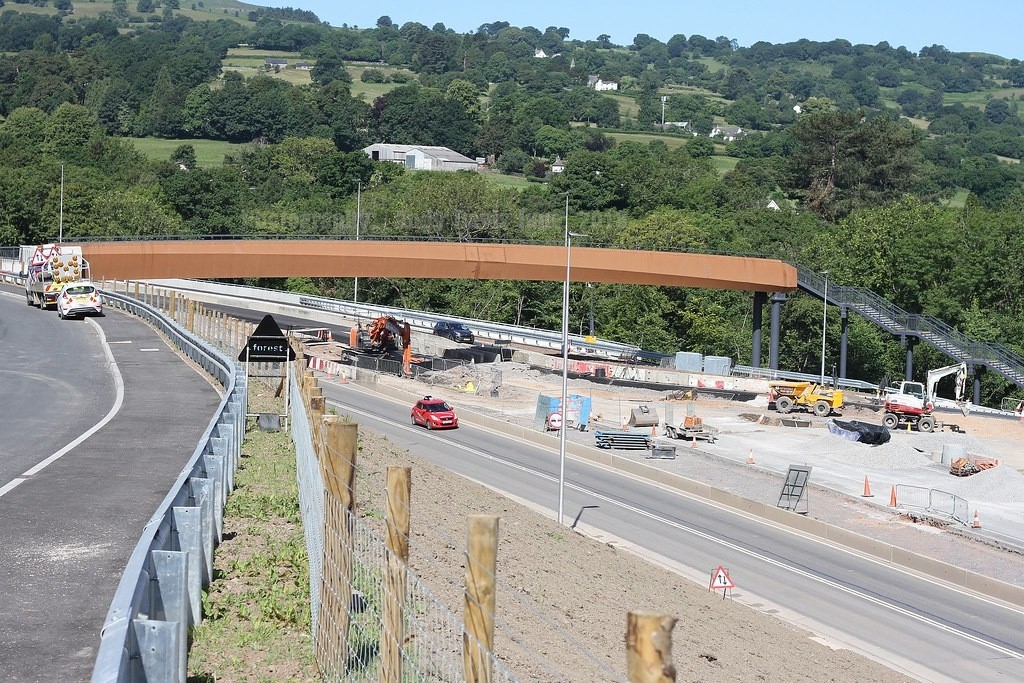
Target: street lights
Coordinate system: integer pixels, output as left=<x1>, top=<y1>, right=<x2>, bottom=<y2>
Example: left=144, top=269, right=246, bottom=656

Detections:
left=558, top=191, right=568, bottom=247
left=820, top=269, right=828, bottom=385
left=558, top=232, right=588, bottom=524
left=352, top=179, right=360, bottom=240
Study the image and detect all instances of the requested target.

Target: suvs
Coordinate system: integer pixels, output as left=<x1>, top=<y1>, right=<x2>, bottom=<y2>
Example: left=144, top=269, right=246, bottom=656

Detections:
left=432, top=320, right=475, bottom=344
left=56, top=283, right=103, bottom=319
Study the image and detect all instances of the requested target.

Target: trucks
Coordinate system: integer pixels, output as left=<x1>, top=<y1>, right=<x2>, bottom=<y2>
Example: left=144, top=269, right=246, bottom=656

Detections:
left=19, top=245, right=83, bottom=311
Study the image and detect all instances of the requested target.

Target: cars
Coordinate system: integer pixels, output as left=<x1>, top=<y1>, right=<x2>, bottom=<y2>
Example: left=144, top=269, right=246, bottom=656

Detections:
left=411, top=399, right=459, bottom=430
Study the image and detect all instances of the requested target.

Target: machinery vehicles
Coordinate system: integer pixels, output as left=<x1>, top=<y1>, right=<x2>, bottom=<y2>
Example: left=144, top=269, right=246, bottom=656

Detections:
left=341, top=317, right=423, bottom=380
left=883, top=362, right=973, bottom=432
left=768, top=381, right=843, bottom=417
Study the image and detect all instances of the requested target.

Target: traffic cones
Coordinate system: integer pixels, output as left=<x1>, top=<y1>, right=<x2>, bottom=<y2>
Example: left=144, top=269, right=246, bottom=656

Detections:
left=745, top=449, right=755, bottom=464
left=1014, top=401, right=1023, bottom=412
left=887, top=486, right=896, bottom=508
left=971, top=510, right=982, bottom=529
left=622, top=417, right=628, bottom=430
left=861, top=476, right=874, bottom=497
left=690, top=434, right=698, bottom=448
left=1019, top=406, right=1024, bottom=421
left=650, top=424, right=657, bottom=437
